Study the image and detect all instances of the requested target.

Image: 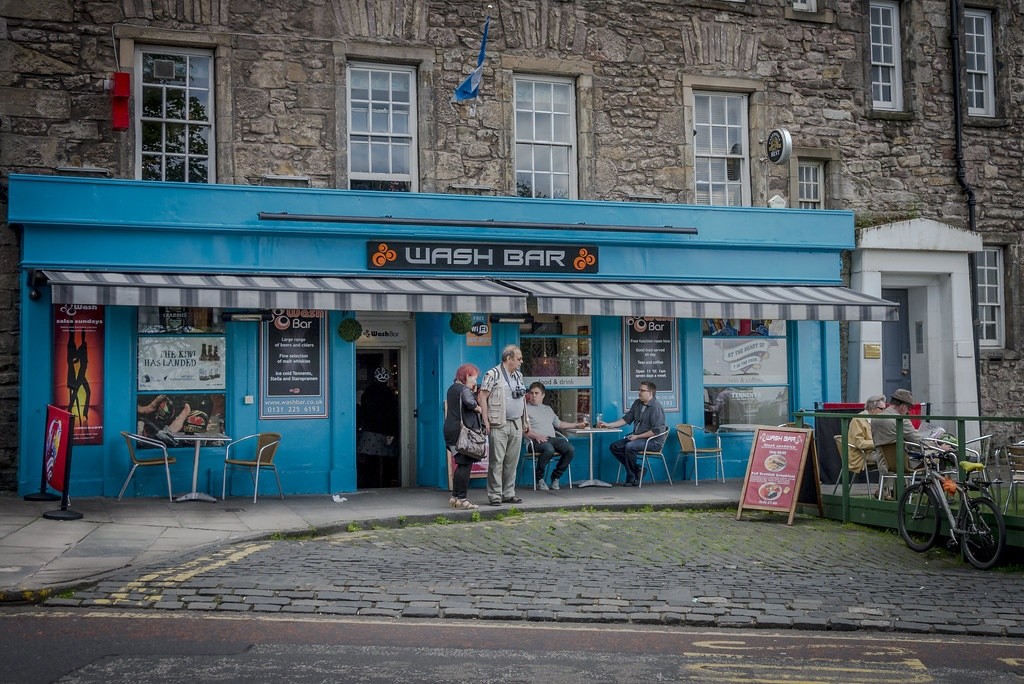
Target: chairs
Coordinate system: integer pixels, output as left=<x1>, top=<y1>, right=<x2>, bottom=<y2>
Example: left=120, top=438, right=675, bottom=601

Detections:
left=778, top=423, right=1024, bottom=516
left=615, top=425, right=673, bottom=488
left=673, top=423, right=726, bottom=487
left=523, top=431, right=573, bottom=491
left=117, top=430, right=176, bottom=502
left=222, top=432, right=284, bottom=504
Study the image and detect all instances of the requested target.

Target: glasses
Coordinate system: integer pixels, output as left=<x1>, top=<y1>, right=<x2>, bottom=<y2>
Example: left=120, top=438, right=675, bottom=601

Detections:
left=510, top=356, right=524, bottom=362
left=637, top=388, right=650, bottom=392
left=876, top=406, right=887, bottom=411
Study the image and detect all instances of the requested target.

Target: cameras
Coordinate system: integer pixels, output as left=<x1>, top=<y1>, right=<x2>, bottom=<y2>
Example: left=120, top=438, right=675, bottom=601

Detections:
left=512, top=389, right=527, bottom=399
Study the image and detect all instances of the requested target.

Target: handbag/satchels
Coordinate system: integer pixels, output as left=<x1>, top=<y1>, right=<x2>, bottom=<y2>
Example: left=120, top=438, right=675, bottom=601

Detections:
left=456, top=423, right=486, bottom=460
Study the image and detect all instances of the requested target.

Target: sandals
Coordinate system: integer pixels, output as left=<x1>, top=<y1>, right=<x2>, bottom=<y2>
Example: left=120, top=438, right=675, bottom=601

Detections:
left=455, top=499, right=478, bottom=509
left=449, top=496, right=458, bottom=507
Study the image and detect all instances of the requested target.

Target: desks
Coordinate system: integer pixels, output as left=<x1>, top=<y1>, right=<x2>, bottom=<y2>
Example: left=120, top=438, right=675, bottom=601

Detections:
left=563, top=427, right=623, bottom=488
left=164, top=431, right=233, bottom=503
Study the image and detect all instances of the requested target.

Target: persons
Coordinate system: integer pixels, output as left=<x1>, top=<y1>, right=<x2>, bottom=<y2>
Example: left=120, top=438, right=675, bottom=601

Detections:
left=357, top=366, right=399, bottom=487
left=596, top=381, right=665, bottom=487
left=710, top=386, right=745, bottom=424
left=848, top=394, right=893, bottom=501
left=523, top=382, right=590, bottom=490
left=478, top=344, right=531, bottom=505
left=443, top=364, right=482, bottom=509
left=872, top=389, right=985, bottom=478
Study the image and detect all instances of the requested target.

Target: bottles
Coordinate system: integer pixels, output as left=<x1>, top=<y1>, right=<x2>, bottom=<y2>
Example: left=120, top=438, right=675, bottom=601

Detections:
left=208, top=345, right=215, bottom=379
left=184, top=394, right=213, bottom=434
left=214, top=346, right=221, bottom=377
left=198, top=344, right=209, bottom=381
left=153, top=394, right=183, bottom=429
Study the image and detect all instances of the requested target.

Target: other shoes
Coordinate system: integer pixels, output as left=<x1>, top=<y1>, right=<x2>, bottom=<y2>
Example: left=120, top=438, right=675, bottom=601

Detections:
left=551, top=479, right=560, bottom=489
left=502, top=496, right=522, bottom=503
left=623, top=481, right=634, bottom=487
left=634, top=468, right=647, bottom=486
left=537, top=479, right=549, bottom=490
left=873, top=489, right=894, bottom=501
left=490, top=498, right=501, bottom=505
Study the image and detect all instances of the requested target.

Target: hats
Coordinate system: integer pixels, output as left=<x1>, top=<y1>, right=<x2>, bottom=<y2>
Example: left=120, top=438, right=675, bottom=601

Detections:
left=890, top=388, right=914, bottom=406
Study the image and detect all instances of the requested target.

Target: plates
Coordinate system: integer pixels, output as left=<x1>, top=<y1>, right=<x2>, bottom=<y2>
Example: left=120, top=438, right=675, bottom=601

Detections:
left=764, top=455, right=787, bottom=473
left=758, top=482, right=782, bottom=501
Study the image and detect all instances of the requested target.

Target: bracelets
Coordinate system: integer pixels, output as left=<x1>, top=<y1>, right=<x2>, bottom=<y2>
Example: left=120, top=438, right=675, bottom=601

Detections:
left=526, top=418, right=529, bottom=421
left=576, top=423, right=578, bottom=429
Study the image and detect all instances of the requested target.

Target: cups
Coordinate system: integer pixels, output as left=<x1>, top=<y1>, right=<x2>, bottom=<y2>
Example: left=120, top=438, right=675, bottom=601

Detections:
left=595, top=414, right=602, bottom=429
left=583, top=414, right=590, bottom=431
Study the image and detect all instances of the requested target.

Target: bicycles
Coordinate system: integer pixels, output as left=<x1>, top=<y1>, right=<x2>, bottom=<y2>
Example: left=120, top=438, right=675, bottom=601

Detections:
left=896, top=442, right=1007, bottom=571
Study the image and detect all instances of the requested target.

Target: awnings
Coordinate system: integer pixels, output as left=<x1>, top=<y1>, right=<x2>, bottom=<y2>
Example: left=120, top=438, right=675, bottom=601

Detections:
left=43, top=272, right=528, bottom=313
left=501, top=280, right=900, bottom=321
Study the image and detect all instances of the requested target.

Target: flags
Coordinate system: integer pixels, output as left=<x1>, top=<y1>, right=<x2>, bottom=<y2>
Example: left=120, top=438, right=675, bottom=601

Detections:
left=455, top=16, right=489, bottom=102
